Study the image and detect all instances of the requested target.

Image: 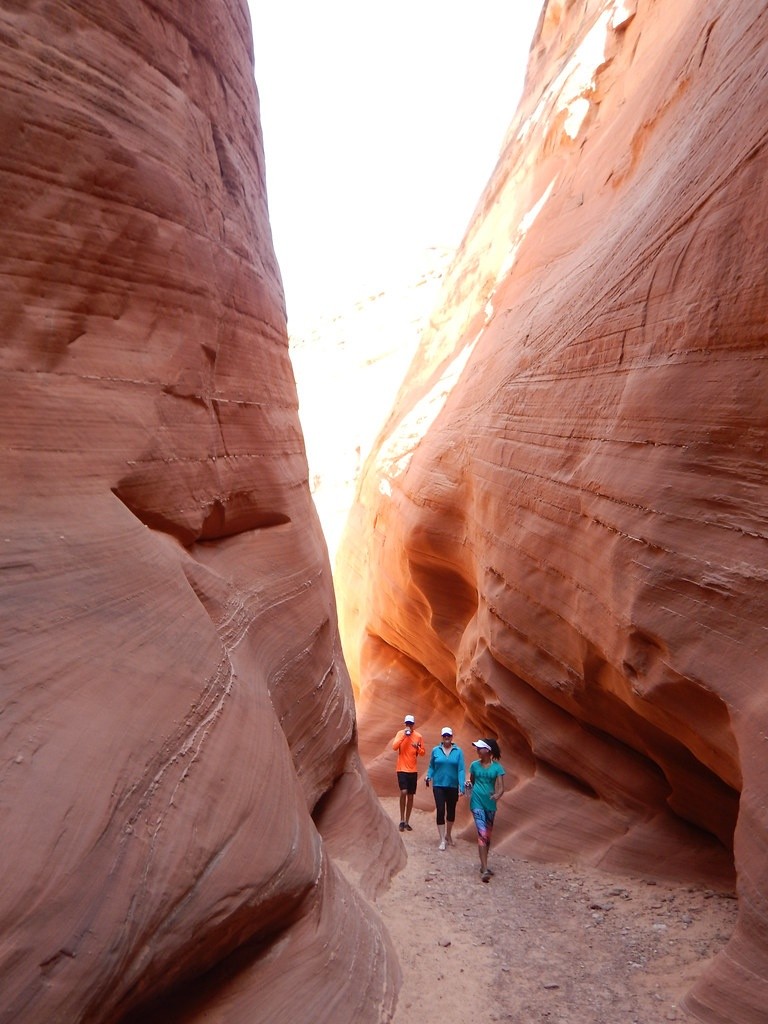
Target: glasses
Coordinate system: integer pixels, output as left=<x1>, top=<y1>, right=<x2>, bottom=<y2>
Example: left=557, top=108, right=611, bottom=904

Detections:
left=442, top=734, right=452, bottom=737
left=406, top=722, right=414, bottom=725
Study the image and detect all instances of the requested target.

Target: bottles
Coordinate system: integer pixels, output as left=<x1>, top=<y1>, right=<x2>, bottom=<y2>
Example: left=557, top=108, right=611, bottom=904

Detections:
left=406, top=730, right=411, bottom=736
left=426, top=775, right=429, bottom=787
left=465, top=780, right=472, bottom=798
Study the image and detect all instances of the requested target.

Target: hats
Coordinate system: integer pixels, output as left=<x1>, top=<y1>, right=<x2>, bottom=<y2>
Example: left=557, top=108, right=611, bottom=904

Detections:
left=405, top=715, right=414, bottom=722
left=472, top=740, right=492, bottom=751
left=441, top=727, right=452, bottom=735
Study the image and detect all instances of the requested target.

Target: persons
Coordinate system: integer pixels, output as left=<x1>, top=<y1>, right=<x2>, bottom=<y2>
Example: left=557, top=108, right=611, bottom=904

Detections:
left=392, top=714, right=425, bottom=832
left=464, top=738, right=506, bottom=881
left=423, top=726, right=466, bottom=851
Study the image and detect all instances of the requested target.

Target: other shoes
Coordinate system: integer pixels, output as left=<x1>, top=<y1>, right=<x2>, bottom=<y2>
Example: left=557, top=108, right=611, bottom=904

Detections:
left=481, top=872, right=490, bottom=883
left=404, top=825, right=412, bottom=831
left=445, top=835, right=455, bottom=846
left=480, top=868, right=494, bottom=875
left=439, top=840, right=447, bottom=850
left=399, top=822, right=405, bottom=831
